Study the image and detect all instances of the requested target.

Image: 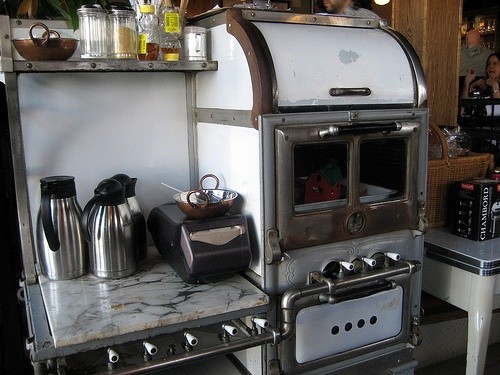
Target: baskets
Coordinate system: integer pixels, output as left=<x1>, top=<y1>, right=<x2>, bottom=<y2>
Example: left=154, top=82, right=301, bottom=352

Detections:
left=426, top=121, right=492, bottom=229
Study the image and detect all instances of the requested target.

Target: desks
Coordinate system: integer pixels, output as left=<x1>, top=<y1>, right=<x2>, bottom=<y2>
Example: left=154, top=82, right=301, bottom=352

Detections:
left=421, top=223, right=500, bottom=375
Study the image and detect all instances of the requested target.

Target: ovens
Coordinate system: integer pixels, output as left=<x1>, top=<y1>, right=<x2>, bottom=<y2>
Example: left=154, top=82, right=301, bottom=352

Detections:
left=194, top=6, right=429, bottom=375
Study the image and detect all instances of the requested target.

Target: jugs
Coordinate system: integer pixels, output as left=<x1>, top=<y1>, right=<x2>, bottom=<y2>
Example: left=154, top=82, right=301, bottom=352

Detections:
left=37, top=176, right=88, bottom=280
left=80, top=178, right=138, bottom=279
left=112, top=173, right=147, bottom=271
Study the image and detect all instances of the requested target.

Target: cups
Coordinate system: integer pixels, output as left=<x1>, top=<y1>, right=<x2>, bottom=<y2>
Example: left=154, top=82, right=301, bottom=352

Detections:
left=184, top=26, right=208, bottom=62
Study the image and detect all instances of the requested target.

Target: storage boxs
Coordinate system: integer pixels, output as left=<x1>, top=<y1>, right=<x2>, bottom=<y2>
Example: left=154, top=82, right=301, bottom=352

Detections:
left=446, top=177, right=500, bottom=242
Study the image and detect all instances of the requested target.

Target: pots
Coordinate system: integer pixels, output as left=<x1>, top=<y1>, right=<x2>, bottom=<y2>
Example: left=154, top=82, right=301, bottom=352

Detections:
left=173, top=175, right=239, bottom=221
left=11, top=21, right=79, bottom=61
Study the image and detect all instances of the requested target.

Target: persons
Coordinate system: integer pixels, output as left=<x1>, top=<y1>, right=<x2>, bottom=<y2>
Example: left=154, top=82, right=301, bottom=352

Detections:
left=321, top=0, right=380, bottom=18
left=458, top=28, right=493, bottom=76
left=461, top=52, right=499, bottom=97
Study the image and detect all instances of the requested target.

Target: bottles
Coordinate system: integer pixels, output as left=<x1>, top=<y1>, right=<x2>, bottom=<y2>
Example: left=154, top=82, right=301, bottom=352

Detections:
left=107, top=6, right=138, bottom=61
left=77, top=4, right=109, bottom=59
left=158, top=7, right=182, bottom=62
left=136, top=5, right=161, bottom=62
